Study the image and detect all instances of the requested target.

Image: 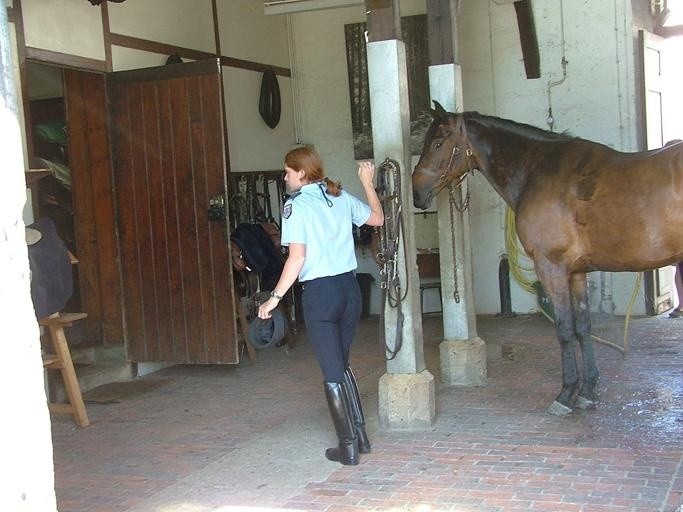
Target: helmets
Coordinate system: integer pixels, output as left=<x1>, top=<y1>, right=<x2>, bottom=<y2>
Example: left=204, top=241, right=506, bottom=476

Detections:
left=248, top=310, right=289, bottom=350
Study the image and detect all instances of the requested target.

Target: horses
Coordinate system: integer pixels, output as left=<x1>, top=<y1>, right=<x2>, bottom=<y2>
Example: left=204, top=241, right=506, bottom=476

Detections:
left=410, top=99, right=683, bottom=418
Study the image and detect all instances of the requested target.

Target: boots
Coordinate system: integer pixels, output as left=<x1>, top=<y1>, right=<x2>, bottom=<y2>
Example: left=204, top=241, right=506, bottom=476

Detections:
left=343, top=366, right=369, bottom=453
left=326, top=380, right=359, bottom=465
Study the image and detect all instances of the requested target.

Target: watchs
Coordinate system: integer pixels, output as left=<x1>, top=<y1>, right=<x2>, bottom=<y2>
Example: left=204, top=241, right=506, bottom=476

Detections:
left=270, top=290, right=284, bottom=300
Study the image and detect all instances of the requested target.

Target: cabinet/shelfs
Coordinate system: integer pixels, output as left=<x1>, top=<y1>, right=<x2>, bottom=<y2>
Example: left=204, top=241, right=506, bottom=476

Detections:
left=24, top=168, right=91, bottom=430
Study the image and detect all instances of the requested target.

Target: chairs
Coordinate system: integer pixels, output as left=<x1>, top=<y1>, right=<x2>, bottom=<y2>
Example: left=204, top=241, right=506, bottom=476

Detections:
left=417, top=248, right=442, bottom=327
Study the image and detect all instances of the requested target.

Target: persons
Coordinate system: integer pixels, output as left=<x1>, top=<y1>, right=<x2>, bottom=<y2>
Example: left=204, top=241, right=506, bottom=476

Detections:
left=257, top=147, right=386, bottom=467
